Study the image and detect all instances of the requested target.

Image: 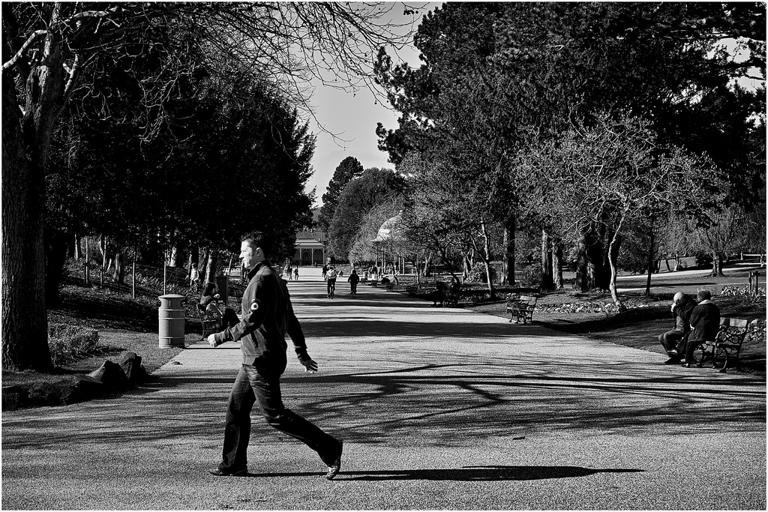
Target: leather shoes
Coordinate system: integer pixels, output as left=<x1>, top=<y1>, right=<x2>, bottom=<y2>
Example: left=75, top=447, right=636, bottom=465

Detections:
left=324, top=438, right=343, bottom=480
left=209, top=465, right=249, bottom=476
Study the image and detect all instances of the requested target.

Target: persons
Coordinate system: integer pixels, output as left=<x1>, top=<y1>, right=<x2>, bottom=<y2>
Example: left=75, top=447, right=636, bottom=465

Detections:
left=348, top=270, right=359, bottom=296
left=656, top=290, right=699, bottom=365
left=184, top=262, right=200, bottom=287
left=293, top=265, right=300, bottom=280
left=205, top=228, right=344, bottom=481
left=198, top=282, right=241, bottom=331
left=665, top=290, right=721, bottom=367
left=287, top=266, right=293, bottom=280
left=368, top=265, right=374, bottom=274
left=321, top=265, right=330, bottom=280
left=323, top=266, right=337, bottom=299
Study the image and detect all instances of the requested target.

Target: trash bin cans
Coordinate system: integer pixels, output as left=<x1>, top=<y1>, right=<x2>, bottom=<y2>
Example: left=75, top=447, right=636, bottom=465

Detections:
left=363, top=271, right=368, bottom=282
left=159, top=293, right=185, bottom=349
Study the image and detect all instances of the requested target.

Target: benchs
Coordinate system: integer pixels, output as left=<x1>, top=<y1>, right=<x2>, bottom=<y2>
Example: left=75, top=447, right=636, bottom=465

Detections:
left=504, top=296, right=537, bottom=324
left=674, top=316, right=747, bottom=370
left=196, top=303, right=219, bottom=339
left=234, top=289, right=242, bottom=314
left=361, top=278, right=458, bottom=307
